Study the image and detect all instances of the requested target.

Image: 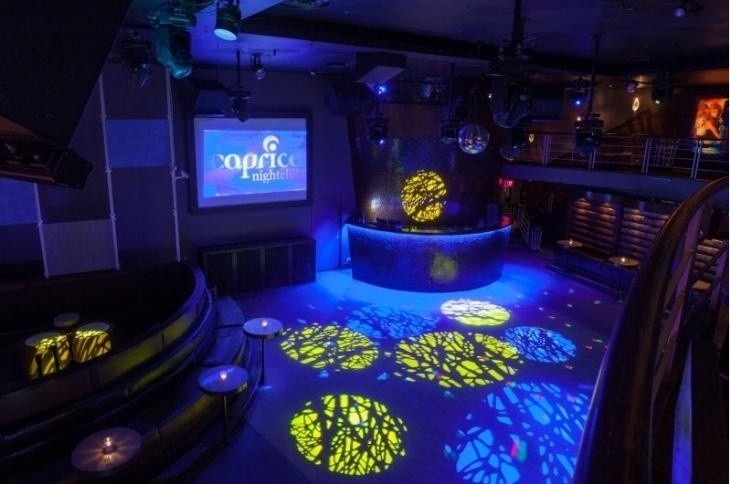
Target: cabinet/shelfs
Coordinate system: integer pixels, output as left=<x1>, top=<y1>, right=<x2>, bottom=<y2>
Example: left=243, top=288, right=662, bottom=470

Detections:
left=198, top=230, right=319, bottom=298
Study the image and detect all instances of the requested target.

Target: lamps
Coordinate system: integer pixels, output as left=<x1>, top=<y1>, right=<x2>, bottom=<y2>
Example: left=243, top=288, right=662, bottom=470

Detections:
left=116, top=0, right=282, bottom=127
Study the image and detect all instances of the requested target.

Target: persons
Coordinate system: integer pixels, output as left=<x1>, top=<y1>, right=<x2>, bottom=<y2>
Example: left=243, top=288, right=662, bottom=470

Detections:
left=695, top=101, right=723, bottom=139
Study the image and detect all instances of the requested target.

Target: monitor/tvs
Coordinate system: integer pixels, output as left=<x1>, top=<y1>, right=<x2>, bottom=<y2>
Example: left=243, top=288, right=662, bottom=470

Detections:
left=187, top=113, right=315, bottom=213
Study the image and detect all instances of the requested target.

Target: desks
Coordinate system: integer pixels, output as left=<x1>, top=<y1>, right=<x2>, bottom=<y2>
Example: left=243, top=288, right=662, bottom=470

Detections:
left=240, top=313, right=282, bottom=388
left=550, top=233, right=728, bottom=305
